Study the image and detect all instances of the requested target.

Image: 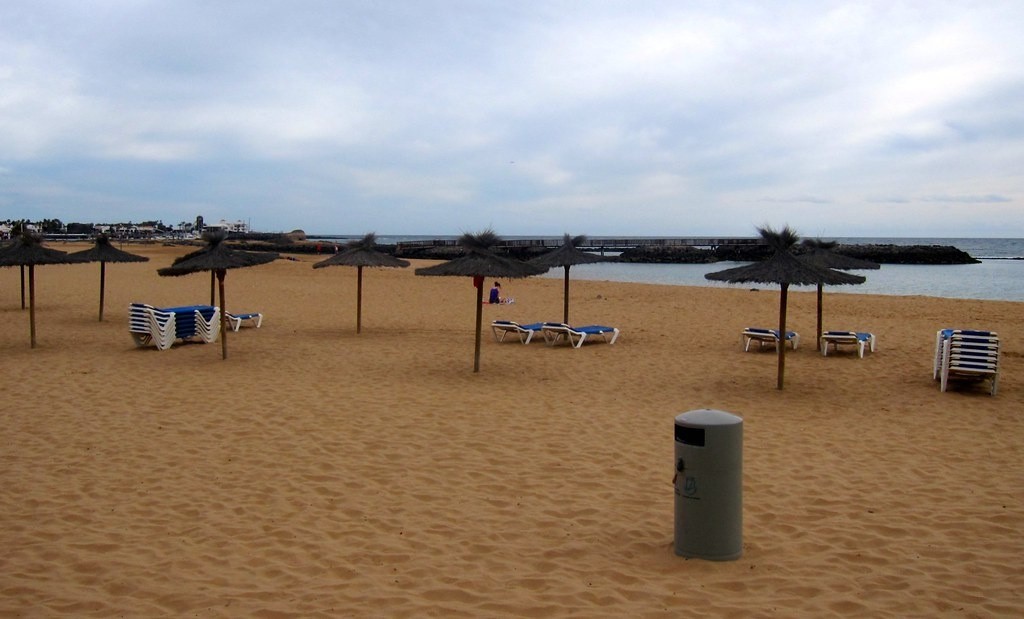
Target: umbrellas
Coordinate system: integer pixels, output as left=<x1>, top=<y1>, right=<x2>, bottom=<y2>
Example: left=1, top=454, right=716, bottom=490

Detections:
left=312, top=230, right=412, bottom=333
left=0, top=232, right=67, bottom=348
left=527, top=239, right=602, bottom=327
left=155, top=226, right=279, bottom=362
left=173, top=239, right=245, bottom=309
left=704, top=224, right=867, bottom=389
left=415, top=230, right=552, bottom=372
left=795, top=238, right=882, bottom=352
left=67, top=231, right=149, bottom=321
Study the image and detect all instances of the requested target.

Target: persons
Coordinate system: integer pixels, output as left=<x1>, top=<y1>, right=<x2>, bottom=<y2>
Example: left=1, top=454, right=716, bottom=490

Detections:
left=489, top=282, right=505, bottom=304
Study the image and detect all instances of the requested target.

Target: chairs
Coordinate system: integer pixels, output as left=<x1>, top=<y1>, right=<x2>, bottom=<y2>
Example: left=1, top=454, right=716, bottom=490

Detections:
left=223, top=310, right=263, bottom=331
left=540, top=321, right=620, bottom=349
left=740, top=328, right=801, bottom=351
left=934, top=328, right=1001, bottom=397
left=491, top=319, right=548, bottom=345
left=821, top=331, right=875, bottom=360
left=127, top=302, right=220, bottom=352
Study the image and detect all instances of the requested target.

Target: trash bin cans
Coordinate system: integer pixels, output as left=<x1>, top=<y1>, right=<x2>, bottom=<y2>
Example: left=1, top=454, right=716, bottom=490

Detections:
left=672, top=408, right=745, bottom=563
